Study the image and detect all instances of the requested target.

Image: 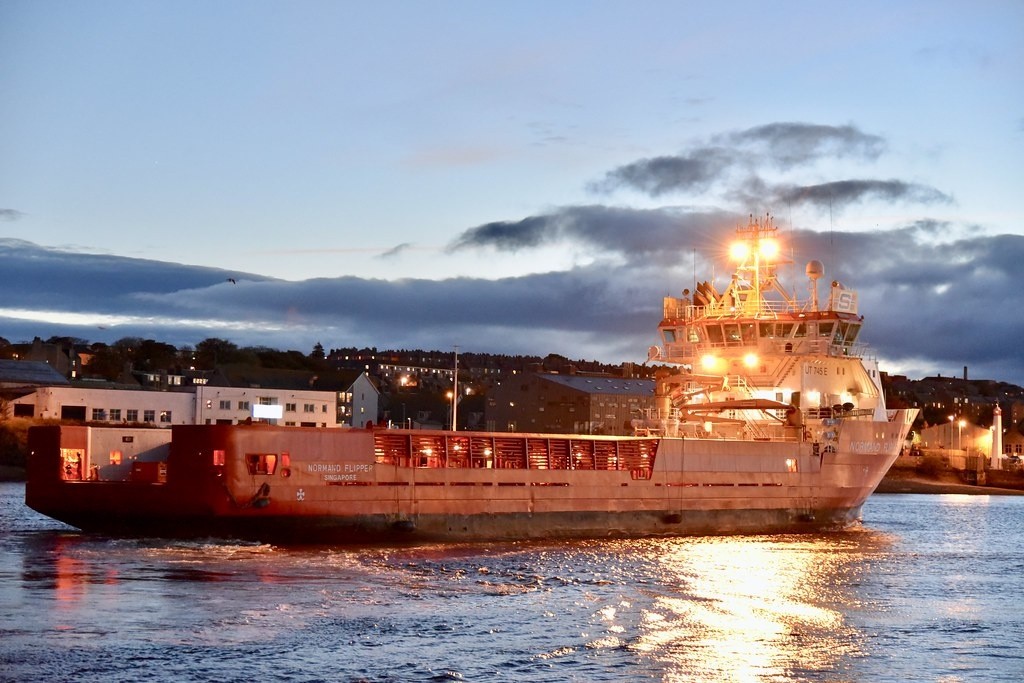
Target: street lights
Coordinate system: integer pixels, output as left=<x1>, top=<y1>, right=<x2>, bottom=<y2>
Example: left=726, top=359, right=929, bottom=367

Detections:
left=958, top=421, right=965, bottom=469
left=948, top=416, right=954, bottom=466
left=446, top=391, right=453, bottom=431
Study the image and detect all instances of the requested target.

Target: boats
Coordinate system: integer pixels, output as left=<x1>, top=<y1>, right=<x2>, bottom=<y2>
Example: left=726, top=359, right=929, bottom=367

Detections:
left=24, top=213, right=920, bottom=540
